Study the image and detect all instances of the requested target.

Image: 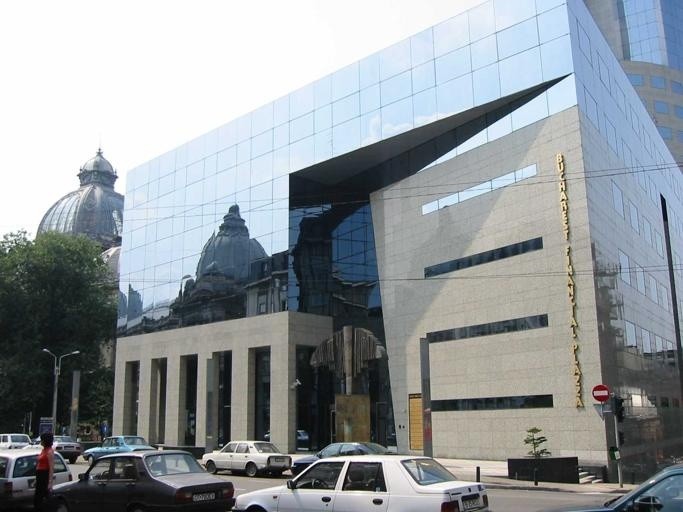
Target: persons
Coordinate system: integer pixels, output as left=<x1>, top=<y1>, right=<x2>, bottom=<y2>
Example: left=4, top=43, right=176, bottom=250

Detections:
left=29, top=432, right=54, bottom=512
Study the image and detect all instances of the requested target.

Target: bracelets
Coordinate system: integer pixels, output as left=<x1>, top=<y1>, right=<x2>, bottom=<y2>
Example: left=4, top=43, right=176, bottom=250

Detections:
left=48, top=480, right=52, bottom=483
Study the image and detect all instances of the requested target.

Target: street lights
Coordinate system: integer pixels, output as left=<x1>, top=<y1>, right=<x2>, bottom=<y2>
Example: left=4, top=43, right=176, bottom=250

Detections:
left=41, top=349, right=80, bottom=434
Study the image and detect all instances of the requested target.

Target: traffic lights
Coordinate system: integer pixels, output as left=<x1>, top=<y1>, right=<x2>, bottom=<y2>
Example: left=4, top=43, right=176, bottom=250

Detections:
left=615, top=397, right=626, bottom=421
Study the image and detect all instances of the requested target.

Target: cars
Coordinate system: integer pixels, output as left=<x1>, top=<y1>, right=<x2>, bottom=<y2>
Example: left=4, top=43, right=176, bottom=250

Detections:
left=263, top=430, right=309, bottom=442
left=538, top=465, right=683, bottom=512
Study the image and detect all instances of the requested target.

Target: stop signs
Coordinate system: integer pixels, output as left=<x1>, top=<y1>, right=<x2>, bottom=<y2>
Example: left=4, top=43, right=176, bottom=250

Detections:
left=592, top=385, right=610, bottom=402
